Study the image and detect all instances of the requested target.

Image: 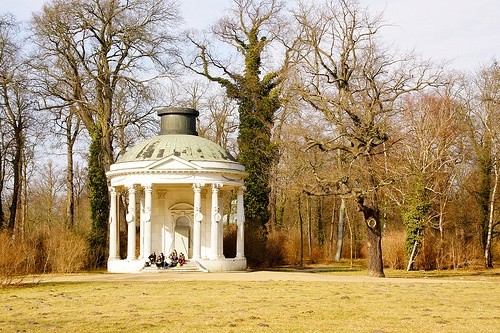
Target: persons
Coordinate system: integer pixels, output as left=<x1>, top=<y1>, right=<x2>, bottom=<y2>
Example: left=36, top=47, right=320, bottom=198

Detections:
left=149, top=249, right=184, bottom=270
